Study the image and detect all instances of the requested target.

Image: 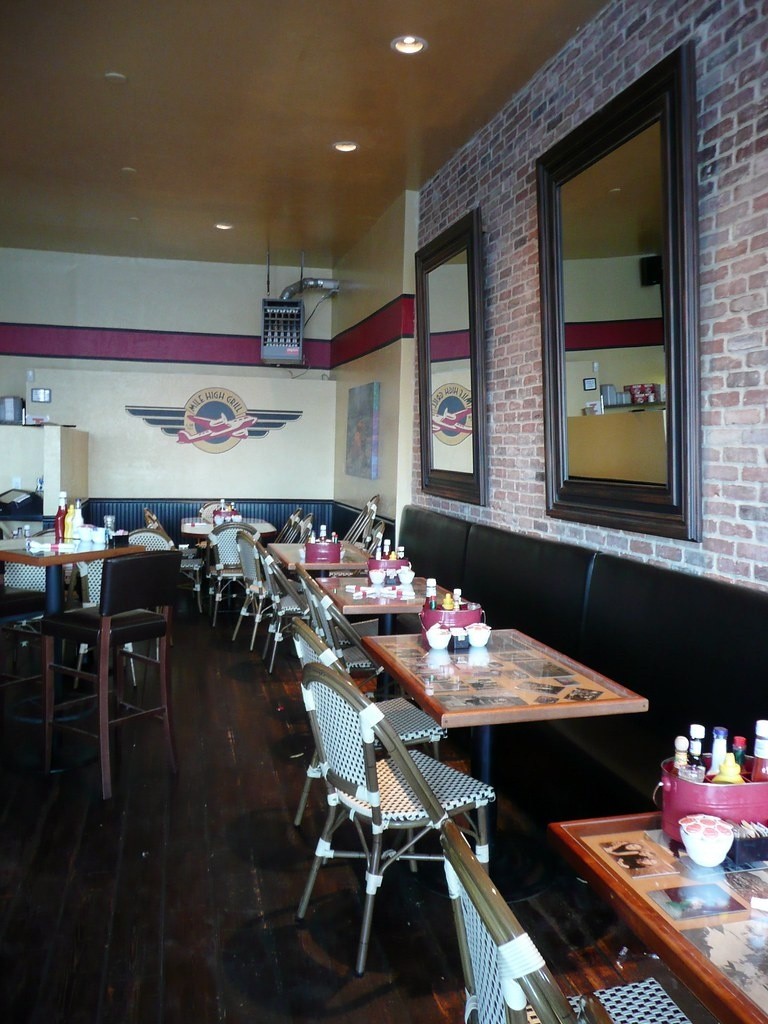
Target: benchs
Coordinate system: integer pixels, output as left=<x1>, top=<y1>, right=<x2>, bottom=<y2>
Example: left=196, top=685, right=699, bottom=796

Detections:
left=398, top=504, right=768, bottom=808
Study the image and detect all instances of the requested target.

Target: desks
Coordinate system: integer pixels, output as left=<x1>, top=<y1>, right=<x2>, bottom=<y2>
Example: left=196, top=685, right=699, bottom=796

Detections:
left=0, top=546, right=146, bottom=724
left=316, top=575, right=474, bottom=614
left=364, top=629, right=649, bottom=903
left=180, top=516, right=278, bottom=590
left=547, top=811, right=768, bottom=1024
left=270, top=542, right=378, bottom=574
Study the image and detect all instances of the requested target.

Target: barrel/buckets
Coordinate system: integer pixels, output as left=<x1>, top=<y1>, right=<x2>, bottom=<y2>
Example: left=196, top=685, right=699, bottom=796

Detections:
left=418, top=608, right=486, bottom=652
left=652, top=752, right=768, bottom=844
left=305, top=540, right=344, bottom=563
left=213, top=509, right=238, bottom=526
left=365, top=557, right=411, bottom=588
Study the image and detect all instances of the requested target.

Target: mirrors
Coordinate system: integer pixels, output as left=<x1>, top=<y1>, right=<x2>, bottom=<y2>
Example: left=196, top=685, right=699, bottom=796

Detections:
left=536, top=39, right=704, bottom=545
left=414, top=207, right=488, bottom=506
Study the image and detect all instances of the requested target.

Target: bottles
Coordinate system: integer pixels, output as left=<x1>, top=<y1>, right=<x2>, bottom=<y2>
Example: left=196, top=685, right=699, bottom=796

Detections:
left=54, top=491, right=84, bottom=557
left=216, top=499, right=237, bottom=512
left=670, top=719, right=768, bottom=785
left=307, top=525, right=339, bottom=544
left=374, top=539, right=406, bottom=560
left=423, top=578, right=480, bottom=611
left=10, top=524, right=31, bottom=552
left=103, top=514, right=115, bottom=547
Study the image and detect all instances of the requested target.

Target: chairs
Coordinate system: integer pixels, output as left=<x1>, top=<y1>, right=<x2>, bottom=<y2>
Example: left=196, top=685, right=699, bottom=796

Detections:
left=290, top=618, right=449, bottom=827
left=125, top=494, right=386, bottom=674
left=296, top=662, right=498, bottom=979
left=440, top=819, right=718, bottom=1024
left=295, top=563, right=384, bottom=687
left=71, top=528, right=175, bottom=692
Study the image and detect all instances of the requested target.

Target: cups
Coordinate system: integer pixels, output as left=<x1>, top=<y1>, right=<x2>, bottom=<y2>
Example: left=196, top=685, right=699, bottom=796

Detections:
left=679, top=826, right=734, bottom=867
left=465, top=628, right=491, bottom=647
left=425, top=631, right=451, bottom=649
left=368, top=571, right=385, bottom=585
left=398, top=571, right=415, bottom=584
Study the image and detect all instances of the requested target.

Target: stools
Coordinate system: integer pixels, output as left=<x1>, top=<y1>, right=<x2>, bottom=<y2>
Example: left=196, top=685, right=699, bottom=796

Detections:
left=41, top=551, right=183, bottom=800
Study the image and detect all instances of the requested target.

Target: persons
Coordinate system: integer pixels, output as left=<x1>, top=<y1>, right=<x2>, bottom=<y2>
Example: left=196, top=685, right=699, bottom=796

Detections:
left=603, top=842, right=656, bottom=869
